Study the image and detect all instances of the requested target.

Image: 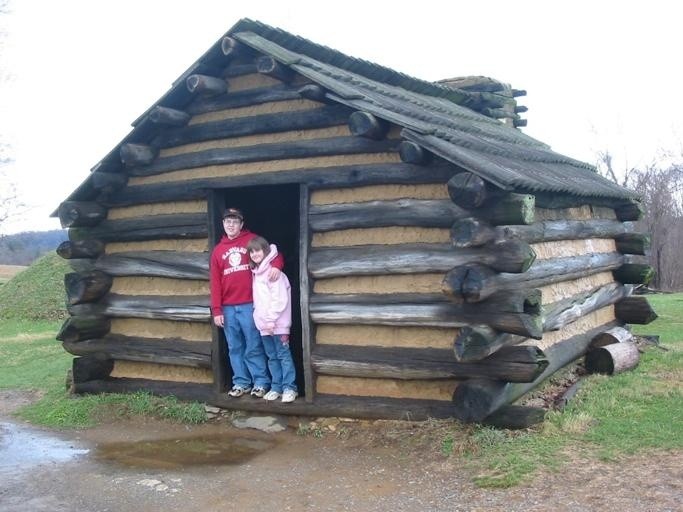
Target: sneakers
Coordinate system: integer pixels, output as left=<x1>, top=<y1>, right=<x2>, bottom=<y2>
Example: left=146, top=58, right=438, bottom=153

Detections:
left=228, top=385, right=298, bottom=402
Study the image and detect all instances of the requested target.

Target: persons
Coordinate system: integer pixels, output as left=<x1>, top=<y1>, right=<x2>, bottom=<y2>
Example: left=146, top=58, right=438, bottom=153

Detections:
left=210, top=207, right=299, bottom=403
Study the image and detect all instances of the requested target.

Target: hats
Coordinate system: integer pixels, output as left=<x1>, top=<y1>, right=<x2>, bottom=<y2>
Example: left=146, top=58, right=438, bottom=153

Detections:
left=223, top=207, right=243, bottom=218
